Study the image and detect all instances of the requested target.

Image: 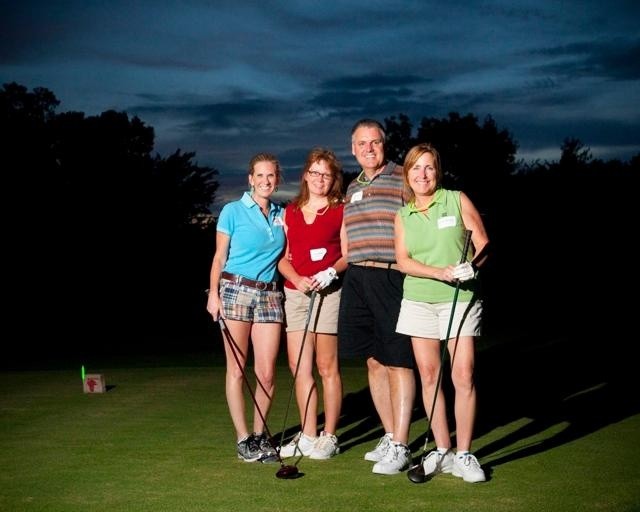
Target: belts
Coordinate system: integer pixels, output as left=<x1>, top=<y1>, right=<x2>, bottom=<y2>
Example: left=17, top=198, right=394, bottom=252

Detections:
left=221, top=270, right=282, bottom=291
left=349, top=258, right=403, bottom=272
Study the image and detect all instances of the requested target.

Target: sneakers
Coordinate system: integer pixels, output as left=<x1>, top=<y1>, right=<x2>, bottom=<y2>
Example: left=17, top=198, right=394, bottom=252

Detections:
left=276, top=431, right=318, bottom=459
left=411, top=451, right=455, bottom=474
left=451, top=454, right=486, bottom=483
left=308, top=431, right=340, bottom=459
left=364, top=434, right=393, bottom=462
left=372, top=443, right=413, bottom=474
left=236, top=431, right=277, bottom=463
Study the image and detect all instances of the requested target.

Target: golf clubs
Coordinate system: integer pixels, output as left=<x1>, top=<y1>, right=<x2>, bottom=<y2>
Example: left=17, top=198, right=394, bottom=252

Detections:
left=259, top=290, right=316, bottom=465
left=206, top=289, right=297, bottom=478
left=407, top=230, right=472, bottom=483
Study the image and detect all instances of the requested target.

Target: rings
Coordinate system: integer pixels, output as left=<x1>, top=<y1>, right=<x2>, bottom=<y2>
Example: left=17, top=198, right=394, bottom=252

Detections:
left=304, top=284, right=308, bottom=288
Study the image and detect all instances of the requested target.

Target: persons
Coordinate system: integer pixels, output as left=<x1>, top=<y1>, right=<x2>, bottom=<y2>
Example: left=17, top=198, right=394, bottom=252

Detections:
left=206, top=153, right=285, bottom=463
left=339, top=119, right=415, bottom=475
left=276, top=148, right=349, bottom=460
left=395, top=145, right=490, bottom=483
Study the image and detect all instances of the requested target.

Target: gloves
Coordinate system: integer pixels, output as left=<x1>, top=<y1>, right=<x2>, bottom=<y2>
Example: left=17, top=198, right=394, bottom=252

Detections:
left=450, top=260, right=477, bottom=283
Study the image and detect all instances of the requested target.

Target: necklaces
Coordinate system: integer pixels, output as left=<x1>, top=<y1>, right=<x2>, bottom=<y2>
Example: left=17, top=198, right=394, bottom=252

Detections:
left=408, top=185, right=442, bottom=212
left=303, top=203, right=329, bottom=216
left=357, top=170, right=369, bottom=184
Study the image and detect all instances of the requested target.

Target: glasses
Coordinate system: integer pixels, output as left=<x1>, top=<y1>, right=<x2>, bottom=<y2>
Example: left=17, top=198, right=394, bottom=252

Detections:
left=306, top=169, right=334, bottom=180
